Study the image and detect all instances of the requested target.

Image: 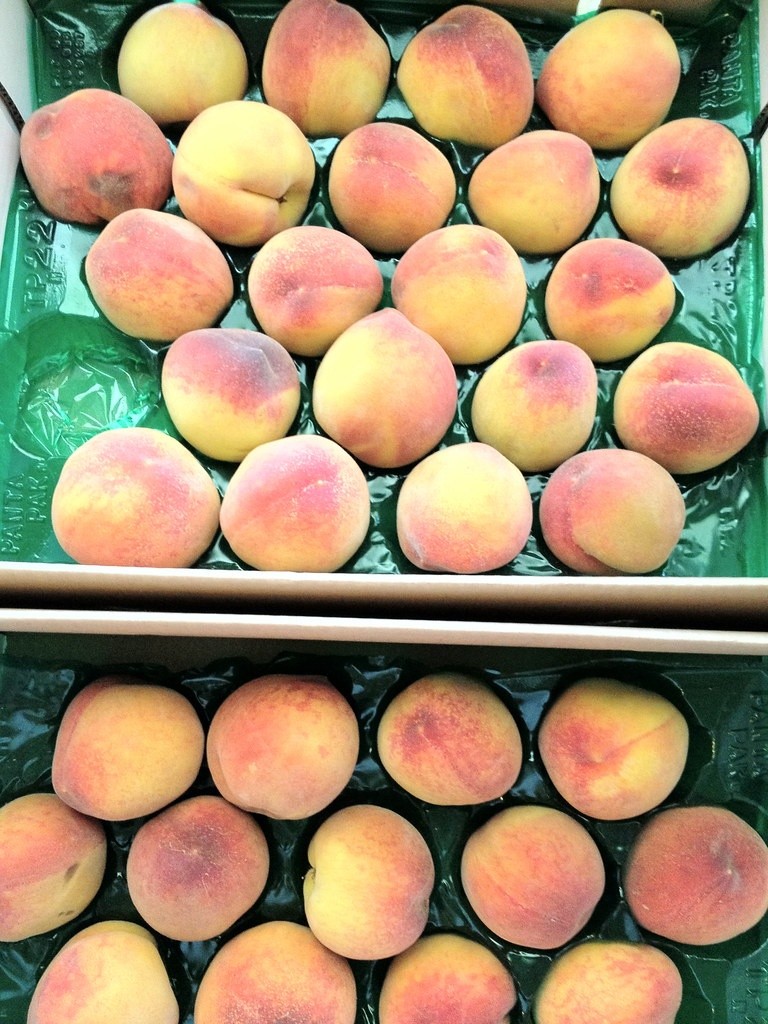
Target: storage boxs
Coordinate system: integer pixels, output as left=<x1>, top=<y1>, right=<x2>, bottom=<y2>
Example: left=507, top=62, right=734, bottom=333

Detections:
left=0, top=0, right=768, bottom=1024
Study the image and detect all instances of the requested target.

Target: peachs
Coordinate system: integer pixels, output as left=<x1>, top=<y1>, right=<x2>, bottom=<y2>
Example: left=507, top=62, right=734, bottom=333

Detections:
left=21, top=0, right=761, bottom=573
left=0, top=672, right=768, bottom=1024
